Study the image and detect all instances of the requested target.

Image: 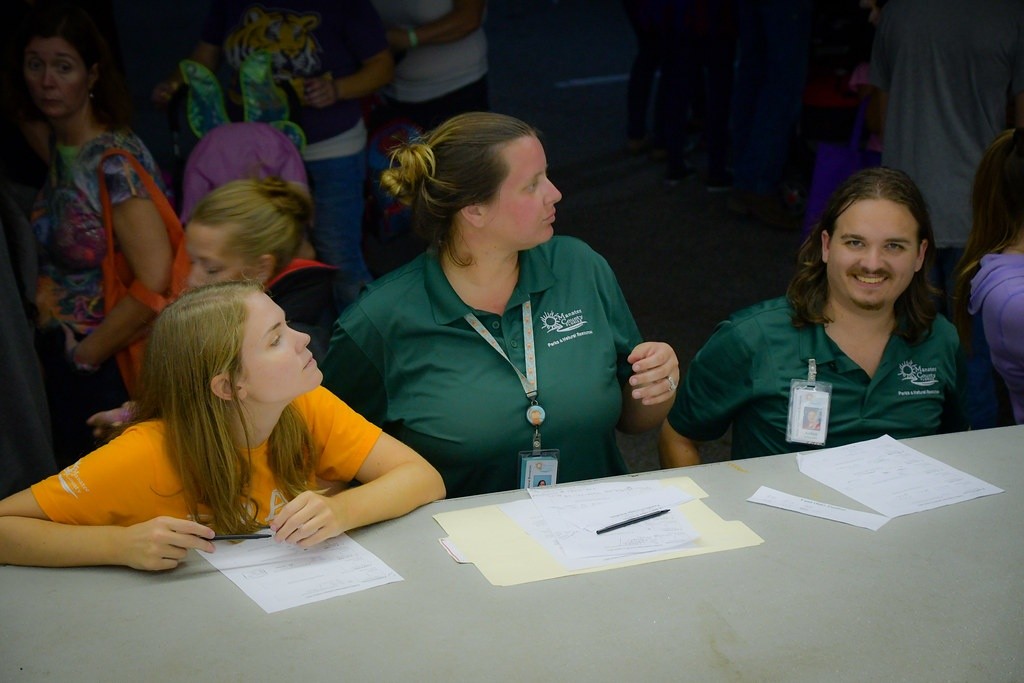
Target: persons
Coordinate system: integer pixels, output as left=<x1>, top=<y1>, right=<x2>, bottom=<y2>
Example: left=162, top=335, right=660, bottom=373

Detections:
left=0, top=281, right=448, bottom=571
left=658, top=166, right=961, bottom=469
left=0, top=0, right=489, bottom=500
left=803, top=409, right=820, bottom=430
left=86, top=176, right=339, bottom=444
left=537, top=480, right=546, bottom=487
left=318, top=110, right=680, bottom=499
left=623, top=0, right=1024, bottom=430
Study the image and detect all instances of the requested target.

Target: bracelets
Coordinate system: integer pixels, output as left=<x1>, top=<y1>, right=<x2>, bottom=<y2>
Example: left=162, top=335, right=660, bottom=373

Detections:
left=70, top=346, right=100, bottom=376
left=328, top=75, right=340, bottom=104
left=407, top=28, right=418, bottom=48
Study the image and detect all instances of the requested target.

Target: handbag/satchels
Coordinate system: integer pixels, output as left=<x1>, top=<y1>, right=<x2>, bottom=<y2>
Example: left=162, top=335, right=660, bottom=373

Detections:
left=800, top=96, right=882, bottom=243
left=99, top=149, right=192, bottom=397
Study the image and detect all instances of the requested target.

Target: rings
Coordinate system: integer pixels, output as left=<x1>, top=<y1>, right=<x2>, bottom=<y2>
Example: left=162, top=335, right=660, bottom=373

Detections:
left=665, top=376, right=678, bottom=392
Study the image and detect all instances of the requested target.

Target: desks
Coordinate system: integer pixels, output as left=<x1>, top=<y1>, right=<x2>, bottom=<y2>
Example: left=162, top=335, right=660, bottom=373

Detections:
left=1, top=421, right=1024, bottom=683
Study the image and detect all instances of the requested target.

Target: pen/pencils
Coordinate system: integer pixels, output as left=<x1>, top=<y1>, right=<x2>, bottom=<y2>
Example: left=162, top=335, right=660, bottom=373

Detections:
left=199, top=533, right=272, bottom=540
left=596, top=509, right=671, bottom=535
